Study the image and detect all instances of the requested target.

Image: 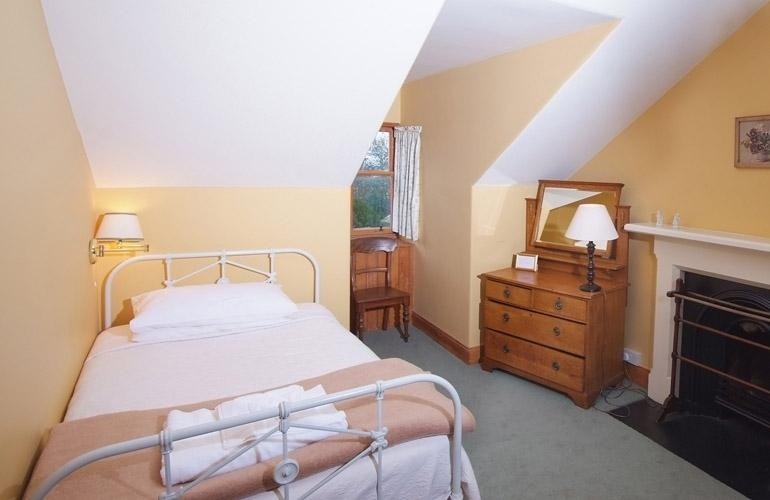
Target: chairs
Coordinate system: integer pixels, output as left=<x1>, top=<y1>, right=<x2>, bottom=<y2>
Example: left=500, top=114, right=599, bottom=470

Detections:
left=350, top=234, right=410, bottom=345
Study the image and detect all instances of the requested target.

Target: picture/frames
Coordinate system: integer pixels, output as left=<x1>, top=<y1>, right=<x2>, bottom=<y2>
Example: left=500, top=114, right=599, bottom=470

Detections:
left=732, top=112, right=769, bottom=174
left=514, top=252, right=539, bottom=271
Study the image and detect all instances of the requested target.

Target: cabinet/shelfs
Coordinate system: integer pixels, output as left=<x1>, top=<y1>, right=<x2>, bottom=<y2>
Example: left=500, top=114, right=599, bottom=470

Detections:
left=474, top=255, right=626, bottom=409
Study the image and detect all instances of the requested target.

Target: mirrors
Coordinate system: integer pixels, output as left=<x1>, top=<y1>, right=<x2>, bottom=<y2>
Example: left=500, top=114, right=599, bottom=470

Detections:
left=529, top=178, right=623, bottom=258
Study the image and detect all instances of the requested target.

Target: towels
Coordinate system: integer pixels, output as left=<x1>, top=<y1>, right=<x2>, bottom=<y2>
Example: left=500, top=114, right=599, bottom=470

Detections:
left=211, top=383, right=314, bottom=449
left=254, top=382, right=349, bottom=462
left=160, top=404, right=256, bottom=488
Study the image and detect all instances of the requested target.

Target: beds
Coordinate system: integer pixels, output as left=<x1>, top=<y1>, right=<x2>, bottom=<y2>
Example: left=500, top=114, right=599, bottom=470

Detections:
left=22, top=248, right=486, bottom=500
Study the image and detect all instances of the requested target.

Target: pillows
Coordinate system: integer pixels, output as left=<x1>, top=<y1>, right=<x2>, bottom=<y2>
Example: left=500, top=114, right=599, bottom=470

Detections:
left=124, top=281, right=298, bottom=331
left=128, top=319, right=298, bottom=343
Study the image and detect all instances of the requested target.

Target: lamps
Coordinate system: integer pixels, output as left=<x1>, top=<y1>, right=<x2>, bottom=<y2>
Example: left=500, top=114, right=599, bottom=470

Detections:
left=87, top=211, right=151, bottom=264
left=564, top=202, right=620, bottom=290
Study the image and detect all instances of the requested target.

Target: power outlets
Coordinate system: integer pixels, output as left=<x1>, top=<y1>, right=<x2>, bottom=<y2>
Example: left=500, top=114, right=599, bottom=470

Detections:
left=621, top=345, right=642, bottom=368
